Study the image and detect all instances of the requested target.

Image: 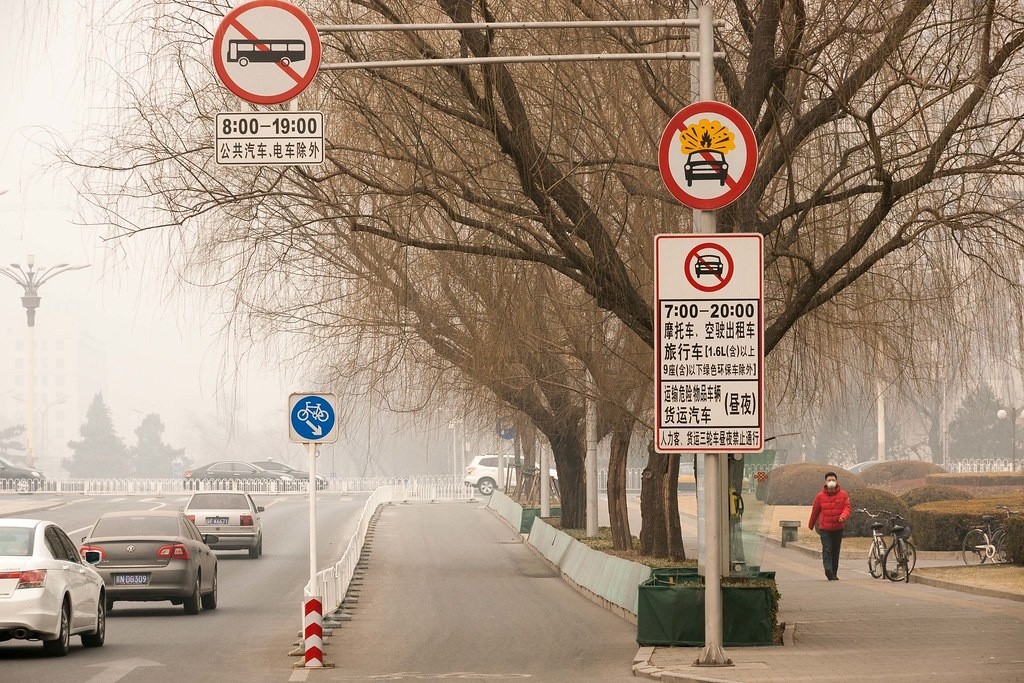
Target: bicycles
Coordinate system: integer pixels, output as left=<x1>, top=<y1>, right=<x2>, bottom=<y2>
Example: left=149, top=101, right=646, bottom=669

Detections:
left=961, top=504, right=1020, bottom=565
left=877, top=509, right=917, bottom=583
left=855, top=507, right=892, bottom=578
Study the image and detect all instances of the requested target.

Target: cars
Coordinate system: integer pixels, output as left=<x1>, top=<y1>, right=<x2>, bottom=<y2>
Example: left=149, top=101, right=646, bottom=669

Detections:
left=0, top=456, right=46, bottom=495
left=250, top=459, right=328, bottom=495
left=79, top=510, right=219, bottom=615
left=675, top=461, right=752, bottom=492
left=179, top=490, right=266, bottom=559
left=184, top=459, right=297, bottom=496
left=846, top=459, right=882, bottom=476
left=0, top=519, right=108, bottom=656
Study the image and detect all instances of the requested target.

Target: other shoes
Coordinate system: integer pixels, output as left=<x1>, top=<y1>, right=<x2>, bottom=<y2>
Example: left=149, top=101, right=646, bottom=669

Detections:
left=825, top=569, right=839, bottom=580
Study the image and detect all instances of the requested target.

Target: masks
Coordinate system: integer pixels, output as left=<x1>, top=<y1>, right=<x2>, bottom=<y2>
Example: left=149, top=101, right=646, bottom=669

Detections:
left=827, top=481, right=835, bottom=489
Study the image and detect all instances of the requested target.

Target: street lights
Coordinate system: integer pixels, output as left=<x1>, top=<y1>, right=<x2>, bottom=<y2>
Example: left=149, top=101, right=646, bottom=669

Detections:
left=998, top=407, right=1024, bottom=475
left=0, top=255, right=93, bottom=467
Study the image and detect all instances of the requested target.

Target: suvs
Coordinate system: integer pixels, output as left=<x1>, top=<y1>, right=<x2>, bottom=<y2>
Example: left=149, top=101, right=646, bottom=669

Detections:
left=463, top=454, right=558, bottom=496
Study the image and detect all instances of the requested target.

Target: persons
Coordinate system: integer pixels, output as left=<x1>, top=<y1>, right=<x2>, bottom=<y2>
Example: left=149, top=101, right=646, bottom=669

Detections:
left=809, top=472, right=851, bottom=580
